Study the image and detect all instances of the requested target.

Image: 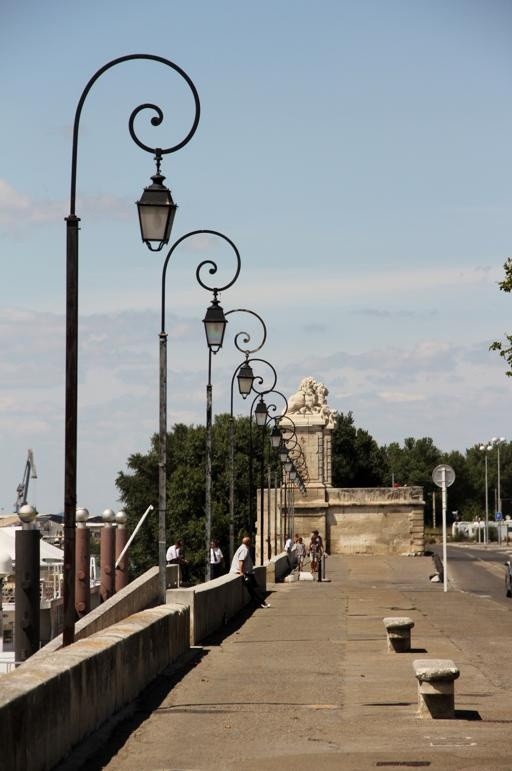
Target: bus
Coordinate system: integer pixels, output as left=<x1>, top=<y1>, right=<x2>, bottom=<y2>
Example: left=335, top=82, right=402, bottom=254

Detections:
left=452, top=520, right=507, bottom=542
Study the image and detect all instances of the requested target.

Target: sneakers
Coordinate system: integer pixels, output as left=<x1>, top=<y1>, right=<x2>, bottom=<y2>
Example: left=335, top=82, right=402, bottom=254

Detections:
left=261, top=599, right=271, bottom=608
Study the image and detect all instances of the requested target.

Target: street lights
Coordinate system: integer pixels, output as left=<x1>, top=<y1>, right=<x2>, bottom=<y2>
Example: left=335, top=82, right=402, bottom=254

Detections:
left=14, top=505, right=130, bottom=662
left=478, top=436, right=507, bottom=542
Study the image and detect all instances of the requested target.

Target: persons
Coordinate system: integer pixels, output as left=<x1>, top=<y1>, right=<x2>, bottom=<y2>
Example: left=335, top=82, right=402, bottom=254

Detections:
left=166, top=540, right=192, bottom=588
left=208, top=538, right=224, bottom=579
left=284, top=530, right=328, bottom=574
left=176, top=539, right=193, bottom=582
left=230, top=536, right=272, bottom=609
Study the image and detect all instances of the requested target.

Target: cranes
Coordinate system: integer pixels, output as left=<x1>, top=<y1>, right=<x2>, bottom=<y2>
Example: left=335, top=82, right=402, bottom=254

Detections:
left=18, top=451, right=37, bottom=511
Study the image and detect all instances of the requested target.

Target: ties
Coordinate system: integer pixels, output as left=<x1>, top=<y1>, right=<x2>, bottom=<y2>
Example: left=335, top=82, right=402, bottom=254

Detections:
left=213, top=551, right=217, bottom=561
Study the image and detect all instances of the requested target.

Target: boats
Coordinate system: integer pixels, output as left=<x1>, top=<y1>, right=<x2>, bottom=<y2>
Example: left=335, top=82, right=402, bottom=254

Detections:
left=1, top=526, right=100, bottom=673
